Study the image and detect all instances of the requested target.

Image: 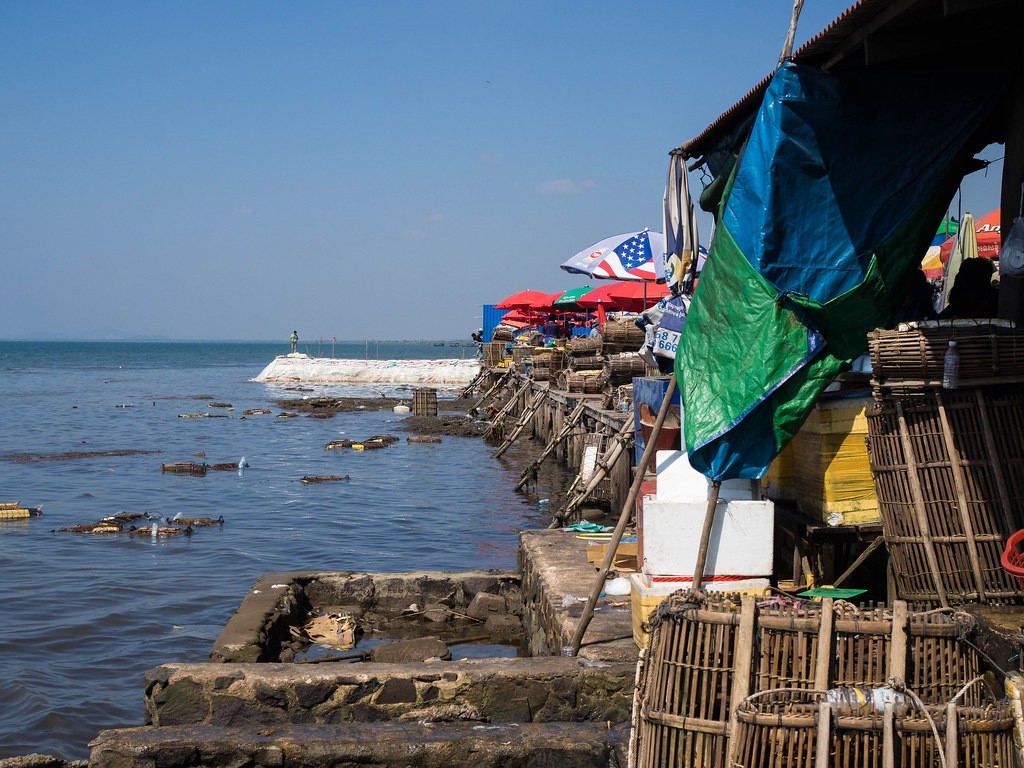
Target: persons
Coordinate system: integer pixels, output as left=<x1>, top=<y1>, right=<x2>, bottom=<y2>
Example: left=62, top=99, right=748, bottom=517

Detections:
left=939, top=257, right=1001, bottom=319
left=537, top=315, right=602, bottom=345
left=471, top=328, right=483, bottom=360
left=290, top=331, right=298, bottom=353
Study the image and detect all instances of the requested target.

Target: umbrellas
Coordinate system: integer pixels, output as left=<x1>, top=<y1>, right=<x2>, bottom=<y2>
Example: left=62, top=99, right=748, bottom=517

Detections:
left=922, top=209, right=1002, bottom=309
left=634, top=150, right=701, bottom=379
left=559, top=228, right=707, bottom=310
left=495, top=281, right=698, bottom=333
